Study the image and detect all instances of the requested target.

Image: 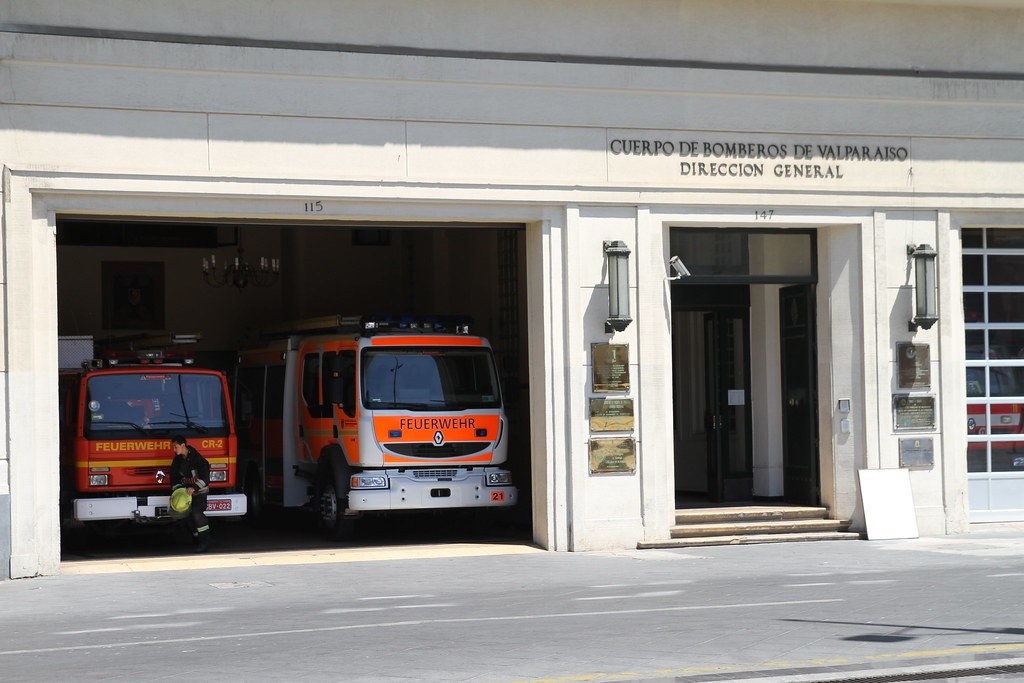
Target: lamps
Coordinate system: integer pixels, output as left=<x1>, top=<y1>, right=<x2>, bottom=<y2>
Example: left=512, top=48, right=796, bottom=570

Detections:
left=907, top=243, right=939, bottom=332
left=603, top=239, right=633, bottom=334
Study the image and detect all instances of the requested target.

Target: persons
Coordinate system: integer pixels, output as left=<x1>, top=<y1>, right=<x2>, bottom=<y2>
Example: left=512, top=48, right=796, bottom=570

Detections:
left=168, top=433, right=214, bottom=553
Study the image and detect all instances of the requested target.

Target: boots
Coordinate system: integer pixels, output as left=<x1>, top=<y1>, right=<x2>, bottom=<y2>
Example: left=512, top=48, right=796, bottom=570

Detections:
left=196, top=530, right=214, bottom=554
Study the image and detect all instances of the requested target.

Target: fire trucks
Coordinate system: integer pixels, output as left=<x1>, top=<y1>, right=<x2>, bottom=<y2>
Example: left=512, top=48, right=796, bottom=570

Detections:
left=219, top=315, right=520, bottom=532
left=58, top=329, right=247, bottom=536
left=961, top=307, right=1024, bottom=450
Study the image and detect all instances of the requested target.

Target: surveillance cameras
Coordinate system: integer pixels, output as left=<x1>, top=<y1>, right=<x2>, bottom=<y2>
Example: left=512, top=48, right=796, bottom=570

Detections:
left=670, top=256, right=691, bottom=280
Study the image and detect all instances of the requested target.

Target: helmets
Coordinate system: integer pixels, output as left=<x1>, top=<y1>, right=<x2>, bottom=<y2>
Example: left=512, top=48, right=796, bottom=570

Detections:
left=171, top=487, right=192, bottom=512
